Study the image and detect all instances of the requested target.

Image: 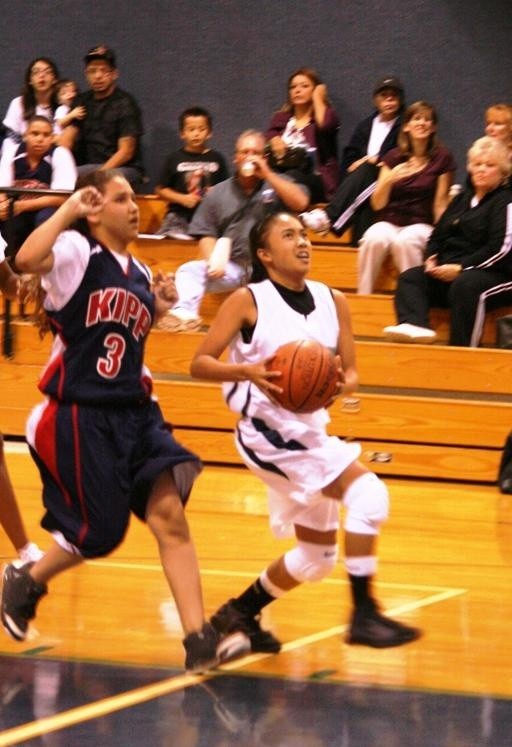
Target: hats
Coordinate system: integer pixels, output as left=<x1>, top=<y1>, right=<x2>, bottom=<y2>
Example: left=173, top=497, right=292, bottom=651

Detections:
left=374, top=76, right=403, bottom=91
left=84, top=45, right=115, bottom=65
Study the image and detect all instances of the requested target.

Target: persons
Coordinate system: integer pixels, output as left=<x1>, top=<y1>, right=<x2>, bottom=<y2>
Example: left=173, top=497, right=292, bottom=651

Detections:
left=152, top=106, right=230, bottom=235
left=0, top=434, right=45, bottom=564
left=296, top=75, right=408, bottom=240
left=187, top=203, right=425, bottom=655
left=381, top=135, right=510, bottom=351
left=2, top=42, right=143, bottom=275
left=352, top=101, right=460, bottom=296
left=2, top=159, right=253, bottom=675
left=265, top=68, right=342, bottom=193
left=462, top=103, right=512, bottom=180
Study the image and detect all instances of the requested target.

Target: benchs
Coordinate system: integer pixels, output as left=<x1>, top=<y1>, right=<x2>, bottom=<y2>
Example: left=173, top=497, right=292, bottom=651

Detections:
left=0, top=193, right=512, bottom=484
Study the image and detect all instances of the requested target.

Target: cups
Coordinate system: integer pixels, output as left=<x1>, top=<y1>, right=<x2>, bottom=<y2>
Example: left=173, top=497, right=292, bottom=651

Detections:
left=210, top=236, right=231, bottom=272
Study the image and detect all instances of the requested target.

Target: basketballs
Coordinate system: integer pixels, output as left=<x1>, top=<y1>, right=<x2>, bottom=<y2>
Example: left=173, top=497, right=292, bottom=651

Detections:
left=267, top=339, right=339, bottom=412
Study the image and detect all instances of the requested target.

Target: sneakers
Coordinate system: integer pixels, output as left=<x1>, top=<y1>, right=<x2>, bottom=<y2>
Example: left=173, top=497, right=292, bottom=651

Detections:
left=383, top=323, right=436, bottom=344
left=1, top=560, right=47, bottom=640
left=180, top=599, right=281, bottom=674
left=345, top=613, right=419, bottom=648
left=157, top=314, right=202, bottom=333
left=298, top=209, right=331, bottom=238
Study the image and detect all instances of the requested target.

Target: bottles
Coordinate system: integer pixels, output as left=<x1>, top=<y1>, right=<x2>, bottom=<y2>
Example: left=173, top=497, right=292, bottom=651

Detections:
left=447, top=183, right=463, bottom=203
left=240, top=160, right=257, bottom=177
